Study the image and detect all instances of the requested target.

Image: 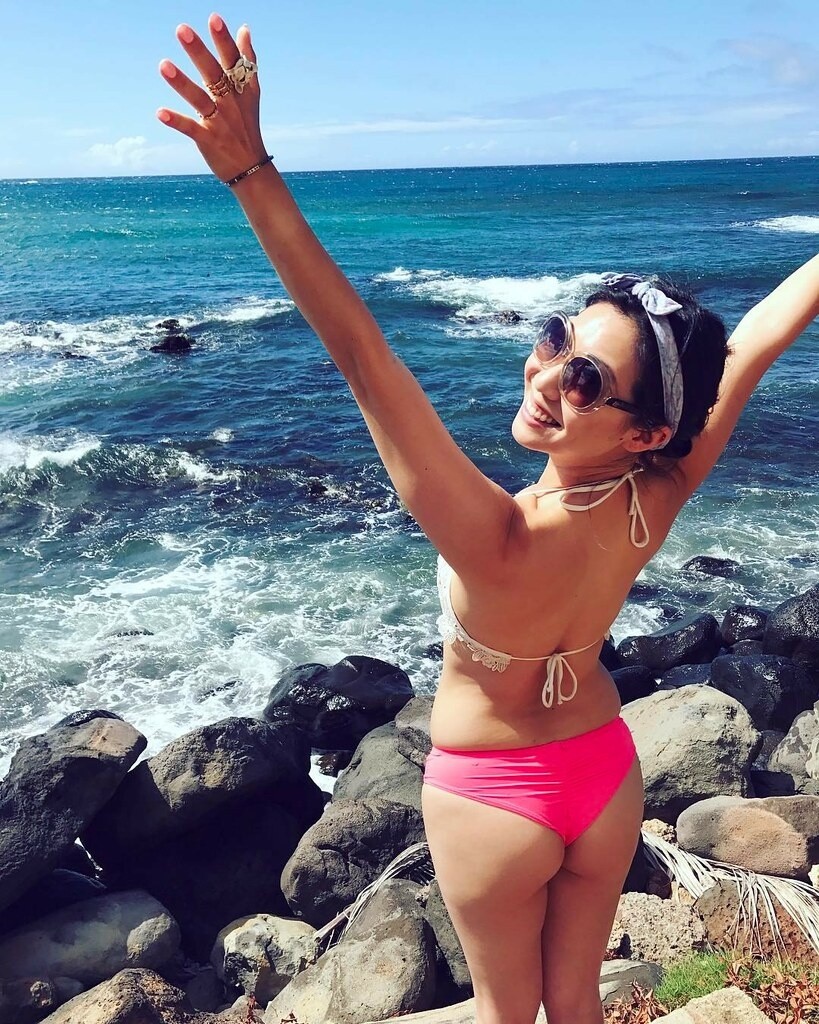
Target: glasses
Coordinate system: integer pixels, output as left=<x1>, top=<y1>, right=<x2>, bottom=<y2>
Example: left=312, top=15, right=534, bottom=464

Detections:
left=533, top=309, right=662, bottom=422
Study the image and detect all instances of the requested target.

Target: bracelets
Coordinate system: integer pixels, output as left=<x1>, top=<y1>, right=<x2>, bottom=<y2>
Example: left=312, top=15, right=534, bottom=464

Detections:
left=223, top=155, right=274, bottom=187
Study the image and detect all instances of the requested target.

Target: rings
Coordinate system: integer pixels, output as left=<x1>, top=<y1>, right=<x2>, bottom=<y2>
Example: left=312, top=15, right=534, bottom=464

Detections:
left=199, top=101, right=219, bottom=121
left=205, top=71, right=235, bottom=97
left=223, top=54, right=259, bottom=95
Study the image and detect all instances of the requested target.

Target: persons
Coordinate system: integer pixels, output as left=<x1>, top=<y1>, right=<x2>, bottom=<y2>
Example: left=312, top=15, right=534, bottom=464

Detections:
left=156, top=9, right=818, bottom=1024
